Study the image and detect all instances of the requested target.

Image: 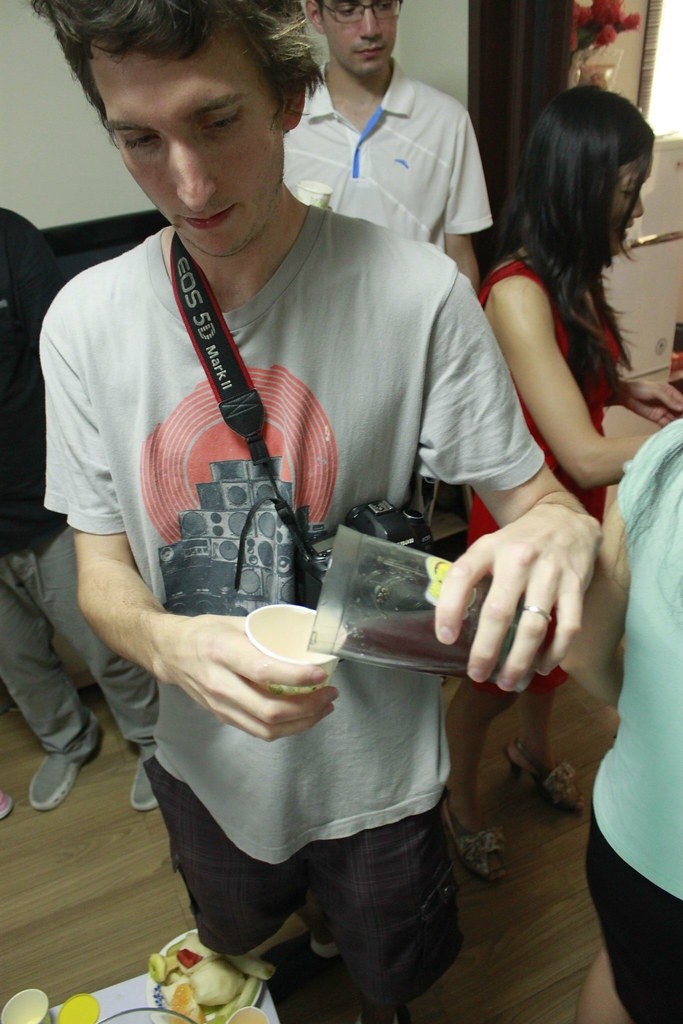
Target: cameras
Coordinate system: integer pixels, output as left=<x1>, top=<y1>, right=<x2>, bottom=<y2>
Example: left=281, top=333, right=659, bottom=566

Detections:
left=292, top=497, right=435, bottom=622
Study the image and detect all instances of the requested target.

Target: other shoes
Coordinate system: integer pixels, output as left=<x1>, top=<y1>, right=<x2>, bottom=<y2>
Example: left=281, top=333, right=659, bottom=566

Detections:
left=260, top=930, right=343, bottom=1003
left=0, top=792, right=13, bottom=819
left=356, top=1004, right=412, bottom=1024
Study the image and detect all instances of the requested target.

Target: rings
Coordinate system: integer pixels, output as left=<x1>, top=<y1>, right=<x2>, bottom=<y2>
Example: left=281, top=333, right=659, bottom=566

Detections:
left=522, top=606, right=555, bottom=624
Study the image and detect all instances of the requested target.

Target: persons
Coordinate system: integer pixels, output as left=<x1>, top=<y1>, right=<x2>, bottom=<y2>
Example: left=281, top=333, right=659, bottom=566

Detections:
left=1, top=0, right=682, bottom=883
left=31, top=0, right=605, bottom=1023
left=560, top=417, right=682, bottom=1024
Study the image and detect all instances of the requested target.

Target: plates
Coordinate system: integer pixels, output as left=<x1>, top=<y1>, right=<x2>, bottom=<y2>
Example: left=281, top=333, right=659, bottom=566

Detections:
left=146, top=929, right=262, bottom=1024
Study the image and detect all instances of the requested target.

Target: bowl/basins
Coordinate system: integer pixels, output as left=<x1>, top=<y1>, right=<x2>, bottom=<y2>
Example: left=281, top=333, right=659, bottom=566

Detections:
left=98, top=1008, right=197, bottom=1024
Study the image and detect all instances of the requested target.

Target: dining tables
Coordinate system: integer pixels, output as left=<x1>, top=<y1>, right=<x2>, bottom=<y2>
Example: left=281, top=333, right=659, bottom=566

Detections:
left=49, top=971, right=280, bottom=1024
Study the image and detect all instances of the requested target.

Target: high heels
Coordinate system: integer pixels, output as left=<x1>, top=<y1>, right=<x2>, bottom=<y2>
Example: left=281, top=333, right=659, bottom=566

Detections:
left=503, top=739, right=582, bottom=814
left=442, top=790, right=514, bottom=885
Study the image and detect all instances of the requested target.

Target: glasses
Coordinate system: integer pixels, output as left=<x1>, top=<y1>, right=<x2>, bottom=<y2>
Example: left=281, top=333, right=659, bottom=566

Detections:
left=316, top=0, right=401, bottom=23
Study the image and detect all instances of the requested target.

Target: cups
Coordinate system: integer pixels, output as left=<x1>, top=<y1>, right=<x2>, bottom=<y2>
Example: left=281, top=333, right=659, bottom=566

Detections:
left=244, top=604, right=346, bottom=696
left=1, top=989, right=51, bottom=1024
left=306, top=525, right=544, bottom=692
left=225, top=1006, right=269, bottom=1024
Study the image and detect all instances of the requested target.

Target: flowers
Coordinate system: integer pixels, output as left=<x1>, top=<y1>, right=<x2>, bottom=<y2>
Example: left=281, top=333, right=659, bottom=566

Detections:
left=571, top=0, right=640, bottom=54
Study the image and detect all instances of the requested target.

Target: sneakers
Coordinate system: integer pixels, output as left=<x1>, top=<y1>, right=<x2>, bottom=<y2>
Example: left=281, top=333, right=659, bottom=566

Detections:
left=130, top=744, right=160, bottom=811
left=29, top=753, right=89, bottom=811
